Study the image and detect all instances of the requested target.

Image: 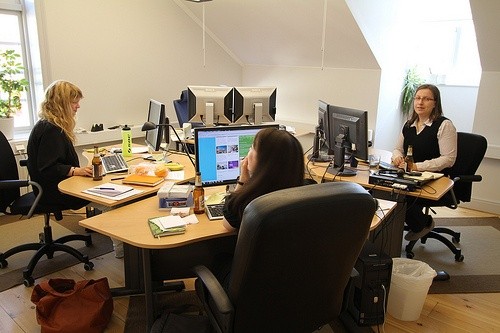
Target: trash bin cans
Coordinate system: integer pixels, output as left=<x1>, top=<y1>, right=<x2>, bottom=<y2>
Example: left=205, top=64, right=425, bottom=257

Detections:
left=387, top=257, right=437, bottom=321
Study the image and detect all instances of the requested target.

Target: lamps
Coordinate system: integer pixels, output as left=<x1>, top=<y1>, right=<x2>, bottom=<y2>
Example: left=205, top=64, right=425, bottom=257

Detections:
left=142, top=121, right=195, bottom=167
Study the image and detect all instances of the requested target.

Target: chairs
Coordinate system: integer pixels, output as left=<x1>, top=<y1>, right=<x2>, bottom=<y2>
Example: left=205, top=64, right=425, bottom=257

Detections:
left=0, top=130, right=95, bottom=288
left=193, top=183, right=377, bottom=333
left=401, top=133, right=487, bottom=259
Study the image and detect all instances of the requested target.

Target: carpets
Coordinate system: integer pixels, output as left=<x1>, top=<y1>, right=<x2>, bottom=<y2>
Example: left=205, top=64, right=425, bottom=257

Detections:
left=0, top=208, right=114, bottom=292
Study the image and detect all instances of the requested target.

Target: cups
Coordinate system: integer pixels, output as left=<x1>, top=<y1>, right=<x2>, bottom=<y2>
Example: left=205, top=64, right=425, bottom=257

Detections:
left=368, top=155, right=380, bottom=174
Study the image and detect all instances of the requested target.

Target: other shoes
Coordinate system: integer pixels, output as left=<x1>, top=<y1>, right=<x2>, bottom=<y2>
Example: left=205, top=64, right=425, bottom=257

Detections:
left=404, top=215, right=435, bottom=240
left=115, top=249, right=124, bottom=258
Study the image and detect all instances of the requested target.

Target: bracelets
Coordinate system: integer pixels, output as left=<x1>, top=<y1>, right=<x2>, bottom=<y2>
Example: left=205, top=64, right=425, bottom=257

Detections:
left=72, top=167, right=76, bottom=176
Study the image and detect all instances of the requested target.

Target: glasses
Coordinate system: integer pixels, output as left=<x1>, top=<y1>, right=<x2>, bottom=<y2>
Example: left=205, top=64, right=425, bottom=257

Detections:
left=414, top=95, right=435, bottom=101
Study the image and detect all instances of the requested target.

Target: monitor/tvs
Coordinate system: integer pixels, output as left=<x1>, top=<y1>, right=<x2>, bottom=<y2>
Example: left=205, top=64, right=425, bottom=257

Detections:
left=144, top=98, right=171, bottom=151
left=307, top=100, right=369, bottom=176
left=187, top=86, right=280, bottom=192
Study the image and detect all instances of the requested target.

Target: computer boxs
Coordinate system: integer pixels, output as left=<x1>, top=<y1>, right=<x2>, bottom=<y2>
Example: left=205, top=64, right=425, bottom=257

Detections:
left=344, top=237, right=393, bottom=326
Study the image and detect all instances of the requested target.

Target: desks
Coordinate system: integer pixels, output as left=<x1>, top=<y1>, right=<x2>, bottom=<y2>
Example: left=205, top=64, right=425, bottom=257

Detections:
left=58, top=144, right=455, bottom=333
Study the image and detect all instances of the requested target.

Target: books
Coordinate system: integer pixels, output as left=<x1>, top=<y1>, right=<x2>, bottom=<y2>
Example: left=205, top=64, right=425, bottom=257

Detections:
left=148, top=215, right=186, bottom=238
left=122, top=174, right=164, bottom=187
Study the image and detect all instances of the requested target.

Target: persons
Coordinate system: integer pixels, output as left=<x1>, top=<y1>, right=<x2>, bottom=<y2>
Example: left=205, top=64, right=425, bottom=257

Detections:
left=27, top=81, right=125, bottom=258
left=391, top=84, right=457, bottom=241
left=222, top=131, right=318, bottom=232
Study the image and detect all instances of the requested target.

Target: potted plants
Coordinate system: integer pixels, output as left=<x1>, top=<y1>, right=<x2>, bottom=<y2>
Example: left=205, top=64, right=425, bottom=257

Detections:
left=0, top=48, right=29, bottom=142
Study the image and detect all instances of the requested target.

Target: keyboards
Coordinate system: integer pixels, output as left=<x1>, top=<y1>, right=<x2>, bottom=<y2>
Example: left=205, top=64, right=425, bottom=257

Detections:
left=204, top=203, right=225, bottom=221
left=378, top=161, right=400, bottom=171
left=101, top=154, right=129, bottom=174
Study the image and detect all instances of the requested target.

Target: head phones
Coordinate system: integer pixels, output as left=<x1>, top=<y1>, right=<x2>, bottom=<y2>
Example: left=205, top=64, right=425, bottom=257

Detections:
left=379, top=168, right=405, bottom=176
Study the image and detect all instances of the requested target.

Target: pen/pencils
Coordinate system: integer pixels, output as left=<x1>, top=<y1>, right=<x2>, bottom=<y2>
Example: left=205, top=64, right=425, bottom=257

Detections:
left=111, top=178, right=124, bottom=180
left=94, top=188, right=114, bottom=190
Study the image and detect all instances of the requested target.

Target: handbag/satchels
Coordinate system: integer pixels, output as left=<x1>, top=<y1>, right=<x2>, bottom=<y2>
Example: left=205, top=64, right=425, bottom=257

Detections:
left=31, top=277, right=114, bottom=333
left=151, top=304, right=213, bottom=333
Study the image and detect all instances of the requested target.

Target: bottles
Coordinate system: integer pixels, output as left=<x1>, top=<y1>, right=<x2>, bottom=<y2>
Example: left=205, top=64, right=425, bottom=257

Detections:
left=405, top=145, right=414, bottom=171
left=193, top=172, right=205, bottom=214
left=92, top=145, right=105, bottom=181
left=122, top=123, right=132, bottom=157
